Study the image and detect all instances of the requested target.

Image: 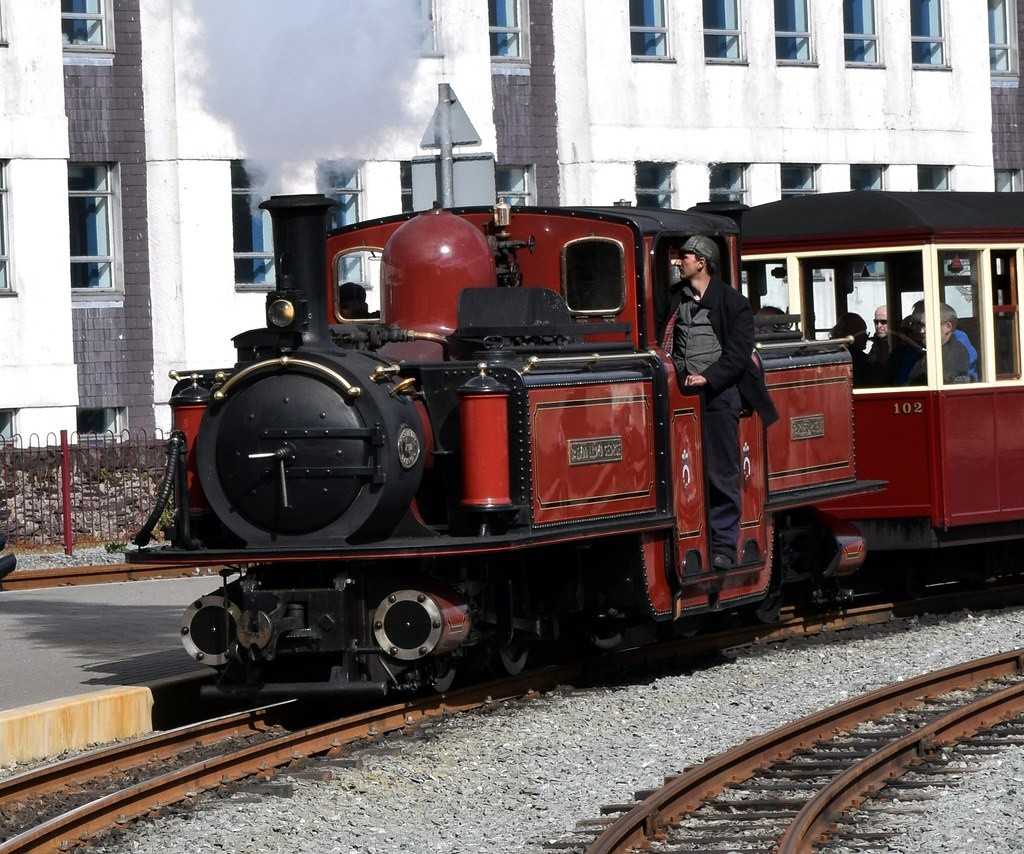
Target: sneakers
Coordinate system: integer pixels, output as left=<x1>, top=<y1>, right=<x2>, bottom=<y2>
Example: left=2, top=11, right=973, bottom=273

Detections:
left=712, top=553, right=732, bottom=570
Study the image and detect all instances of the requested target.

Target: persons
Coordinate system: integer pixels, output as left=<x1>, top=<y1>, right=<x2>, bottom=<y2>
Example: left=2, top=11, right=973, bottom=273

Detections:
left=338, top=283, right=384, bottom=321
left=870, top=306, right=888, bottom=389
left=653, top=235, right=779, bottom=571
left=753, top=307, right=818, bottom=346
left=826, top=313, right=880, bottom=388
left=904, top=301, right=980, bottom=385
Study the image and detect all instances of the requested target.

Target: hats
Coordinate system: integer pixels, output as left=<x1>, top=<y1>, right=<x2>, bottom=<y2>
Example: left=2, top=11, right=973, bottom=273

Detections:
left=681, top=236, right=721, bottom=263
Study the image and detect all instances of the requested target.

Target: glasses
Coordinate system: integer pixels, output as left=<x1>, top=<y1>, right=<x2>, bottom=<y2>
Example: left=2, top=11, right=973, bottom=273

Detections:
left=874, top=319, right=887, bottom=325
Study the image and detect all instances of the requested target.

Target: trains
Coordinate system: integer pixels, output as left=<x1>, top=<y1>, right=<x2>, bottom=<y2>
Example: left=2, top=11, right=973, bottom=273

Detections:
left=126, top=192, right=1024, bottom=699
